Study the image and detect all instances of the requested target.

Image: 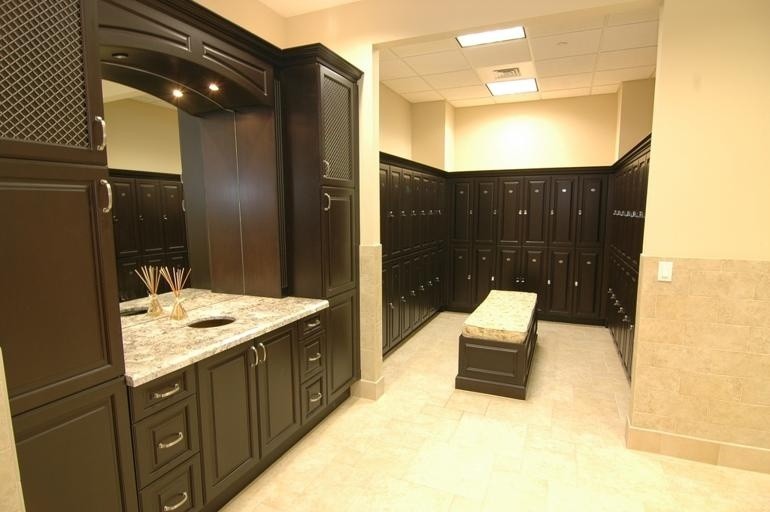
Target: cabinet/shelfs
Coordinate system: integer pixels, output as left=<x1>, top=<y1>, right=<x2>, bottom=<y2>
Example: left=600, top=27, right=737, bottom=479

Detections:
left=377, top=134, right=651, bottom=382
left=1, top=0, right=364, bottom=512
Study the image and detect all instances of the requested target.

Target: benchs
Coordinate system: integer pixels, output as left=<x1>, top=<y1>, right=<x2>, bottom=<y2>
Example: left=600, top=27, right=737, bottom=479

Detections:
left=454, top=289, right=540, bottom=401
